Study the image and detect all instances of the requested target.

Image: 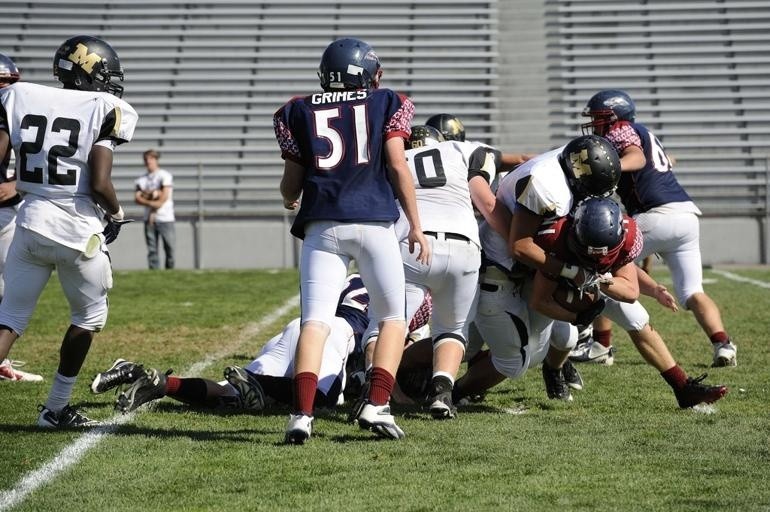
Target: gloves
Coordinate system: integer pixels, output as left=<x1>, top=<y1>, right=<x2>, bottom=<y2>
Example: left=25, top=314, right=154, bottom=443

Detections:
left=560, top=263, right=613, bottom=301
left=101, top=213, right=135, bottom=245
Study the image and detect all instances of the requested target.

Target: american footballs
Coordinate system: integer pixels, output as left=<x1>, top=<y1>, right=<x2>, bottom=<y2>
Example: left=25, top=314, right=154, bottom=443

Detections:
left=553, top=288, right=593, bottom=314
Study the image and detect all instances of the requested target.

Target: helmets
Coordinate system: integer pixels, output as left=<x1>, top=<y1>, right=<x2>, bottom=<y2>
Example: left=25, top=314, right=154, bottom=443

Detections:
left=581, top=91, right=636, bottom=138
left=53, top=36, right=124, bottom=99
left=0, top=54, right=21, bottom=81
left=317, top=39, right=383, bottom=93
left=560, top=135, right=622, bottom=210
left=404, top=124, right=446, bottom=150
left=424, top=114, right=466, bottom=142
left=574, top=196, right=626, bottom=273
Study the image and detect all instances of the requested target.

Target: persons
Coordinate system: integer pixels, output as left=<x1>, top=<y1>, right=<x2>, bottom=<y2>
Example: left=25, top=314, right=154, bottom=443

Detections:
left=270, top=35, right=434, bottom=439
left=0, top=33, right=136, bottom=433
left=0, top=52, right=48, bottom=380
left=567, top=90, right=738, bottom=369
left=88, top=109, right=727, bottom=419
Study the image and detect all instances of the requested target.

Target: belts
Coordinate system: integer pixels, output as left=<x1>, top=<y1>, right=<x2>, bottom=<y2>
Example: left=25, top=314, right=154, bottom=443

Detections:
left=422, top=232, right=473, bottom=242
left=480, top=283, right=521, bottom=295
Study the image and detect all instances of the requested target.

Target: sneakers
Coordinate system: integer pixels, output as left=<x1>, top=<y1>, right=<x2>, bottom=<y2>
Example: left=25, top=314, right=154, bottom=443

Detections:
left=568, top=337, right=616, bottom=366
left=90, top=358, right=143, bottom=395
left=0, top=357, right=44, bottom=384
left=675, top=373, right=728, bottom=409
left=358, top=401, right=406, bottom=439
left=36, top=402, right=105, bottom=430
left=542, top=363, right=573, bottom=403
left=562, top=358, right=584, bottom=390
left=425, top=382, right=459, bottom=420
left=348, top=397, right=368, bottom=424
left=113, top=368, right=174, bottom=413
left=284, top=411, right=315, bottom=444
left=468, top=350, right=485, bottom=402
left=424, top=386, right=461, bottom=405
left=713, top=337, right=738, bottom=365
left=223, top=362, right=268, bottom=413
